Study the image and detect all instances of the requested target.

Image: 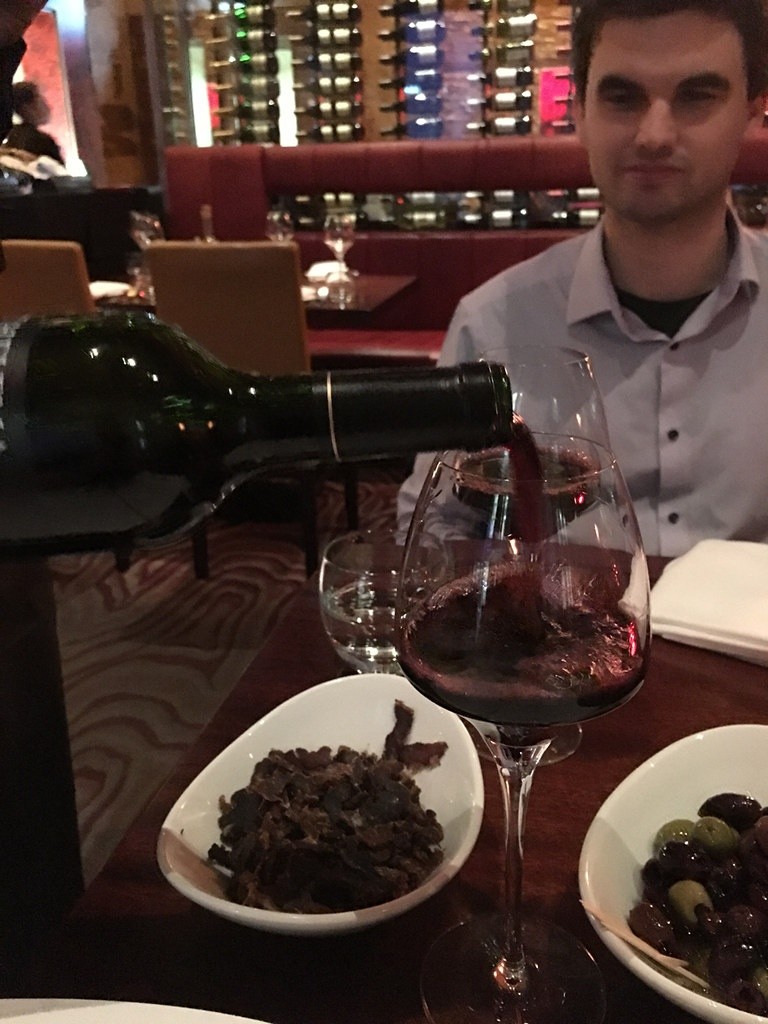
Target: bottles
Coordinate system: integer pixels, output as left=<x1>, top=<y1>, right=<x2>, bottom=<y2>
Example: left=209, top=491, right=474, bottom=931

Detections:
left=552, top=0, right=579, bottom=134
left=291, top=0, right=364, bottom=142
left=377, top=0, right=447, bottom=139
left=0, top=312, right=511, bottom=550
left=199, top=204, right=216, bottom=242
left=207, top=0, right=280, bottom=144
left=468, top=0, right=536, bottom=135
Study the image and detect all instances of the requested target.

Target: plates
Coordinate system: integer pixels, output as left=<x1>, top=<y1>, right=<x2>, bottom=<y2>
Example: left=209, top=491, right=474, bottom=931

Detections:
left=579, top=724, right=768, bottom=1024
left=158, top=676, right=487, bottom=931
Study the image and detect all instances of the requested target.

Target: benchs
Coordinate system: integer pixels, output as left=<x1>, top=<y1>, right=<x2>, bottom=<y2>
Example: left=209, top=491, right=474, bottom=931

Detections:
left=160, top=132, right=768, bottom=370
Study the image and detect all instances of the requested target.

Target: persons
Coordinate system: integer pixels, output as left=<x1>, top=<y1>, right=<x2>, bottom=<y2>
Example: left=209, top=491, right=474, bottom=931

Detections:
left=3, top=81, right=65, bottom=165
left=397, top=0, right=768, bottom=556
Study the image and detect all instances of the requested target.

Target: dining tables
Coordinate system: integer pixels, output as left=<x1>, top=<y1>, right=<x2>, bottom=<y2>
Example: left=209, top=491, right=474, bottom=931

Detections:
left=0, top=539, right=768, bottom=1024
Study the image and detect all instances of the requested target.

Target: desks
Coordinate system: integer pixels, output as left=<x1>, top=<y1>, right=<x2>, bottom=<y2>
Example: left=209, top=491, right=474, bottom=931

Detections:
left=97, top=273, right=419, bottom=327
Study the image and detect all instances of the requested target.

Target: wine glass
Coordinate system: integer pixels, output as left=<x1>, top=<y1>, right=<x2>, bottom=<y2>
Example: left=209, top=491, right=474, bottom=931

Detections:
left=317, top=271, right=356, bottom=304
left=396, top=347, right=628, bottom=769
left=324, top=219, right=357, bottom=262
left=393, top=432, right=612, bottom=1024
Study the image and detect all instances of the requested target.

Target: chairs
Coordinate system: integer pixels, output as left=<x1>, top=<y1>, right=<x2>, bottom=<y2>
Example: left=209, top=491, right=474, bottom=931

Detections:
left=143, top=239, right=359, bottom=583
left=0, top=238, right=94, bottom=321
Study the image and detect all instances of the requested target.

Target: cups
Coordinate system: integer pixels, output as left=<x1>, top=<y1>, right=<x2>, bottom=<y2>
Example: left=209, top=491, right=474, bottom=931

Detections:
left=266, top=212, right=294, bottom=242
left=318, top=530, right=449, bottom=673
